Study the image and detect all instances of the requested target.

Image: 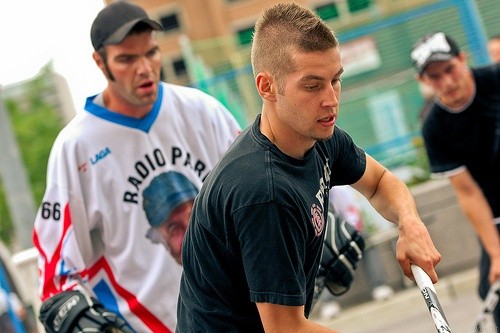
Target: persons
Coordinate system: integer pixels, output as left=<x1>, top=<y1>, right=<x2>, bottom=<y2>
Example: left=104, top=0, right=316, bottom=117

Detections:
left=175, top=3, right=442, bottom=333
left=408, top=29, right=500, bottom=333
left=31, top=2, right=365, bottom=333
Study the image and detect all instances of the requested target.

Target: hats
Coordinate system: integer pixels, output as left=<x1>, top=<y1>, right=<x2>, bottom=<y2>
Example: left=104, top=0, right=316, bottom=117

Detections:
left=409, top=31, right=460, bottom=79
left=90, top=1, right=164, bottom=52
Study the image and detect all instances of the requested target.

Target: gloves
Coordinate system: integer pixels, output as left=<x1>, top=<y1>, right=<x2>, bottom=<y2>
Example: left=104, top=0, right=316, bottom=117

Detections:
left=315, top=202, right=366, bottom=299
left=38, top=288, right=128, bottom=333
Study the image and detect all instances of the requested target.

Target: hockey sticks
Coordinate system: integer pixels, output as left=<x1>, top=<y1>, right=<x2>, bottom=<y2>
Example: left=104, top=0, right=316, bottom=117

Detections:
left=411, top=263, right=453, bottom=333
left=470, top=280, right=500, bottom=333
